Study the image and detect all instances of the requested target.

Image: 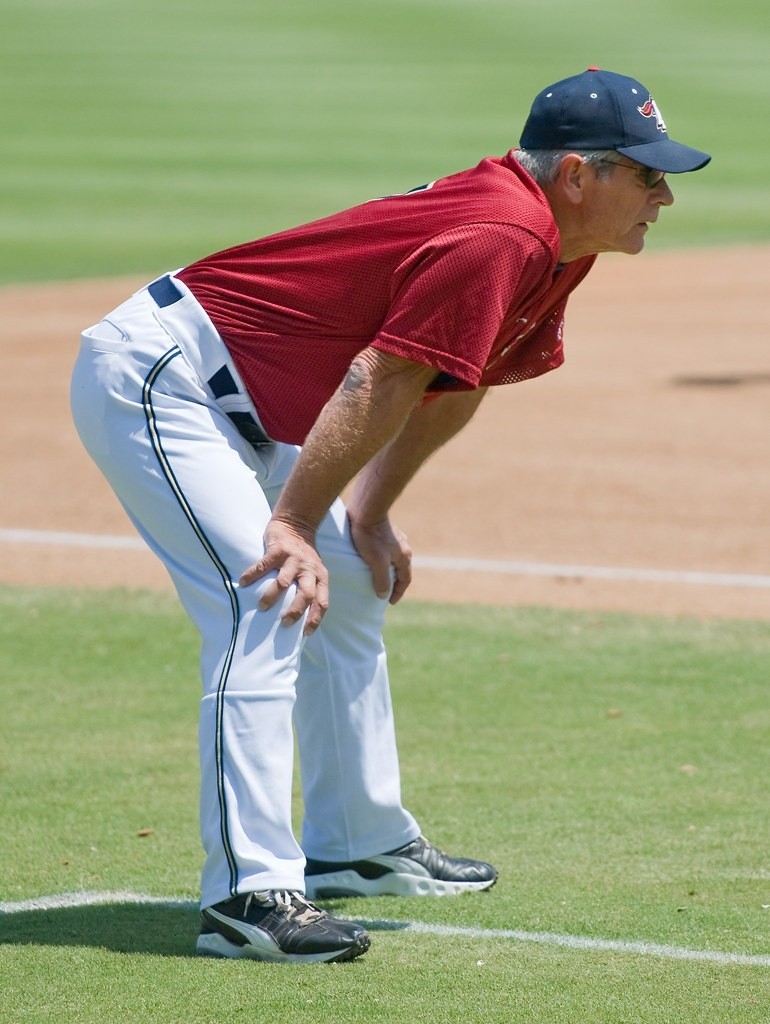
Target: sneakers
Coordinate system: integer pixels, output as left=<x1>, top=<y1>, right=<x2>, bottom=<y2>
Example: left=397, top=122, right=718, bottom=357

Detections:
left=195, top=887, right=370, bottom=965
left=303, top=833, right=497, bottom=899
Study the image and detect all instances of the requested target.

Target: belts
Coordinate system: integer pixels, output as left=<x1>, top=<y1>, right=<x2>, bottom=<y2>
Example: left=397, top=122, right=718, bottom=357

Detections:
left=147, top=276, right=273, bottom=450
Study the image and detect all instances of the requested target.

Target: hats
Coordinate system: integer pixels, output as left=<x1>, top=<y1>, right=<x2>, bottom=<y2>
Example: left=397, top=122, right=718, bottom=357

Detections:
left=519, top=67, right=713, bottom=174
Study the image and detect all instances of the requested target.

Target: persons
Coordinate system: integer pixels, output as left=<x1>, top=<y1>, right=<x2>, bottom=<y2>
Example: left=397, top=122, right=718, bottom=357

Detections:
left=72, top=67, right=714, bottom=960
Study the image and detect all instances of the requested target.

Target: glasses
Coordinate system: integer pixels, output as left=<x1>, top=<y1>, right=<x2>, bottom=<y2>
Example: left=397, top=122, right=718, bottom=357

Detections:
left=591, top=151, right=665, bottom=188
